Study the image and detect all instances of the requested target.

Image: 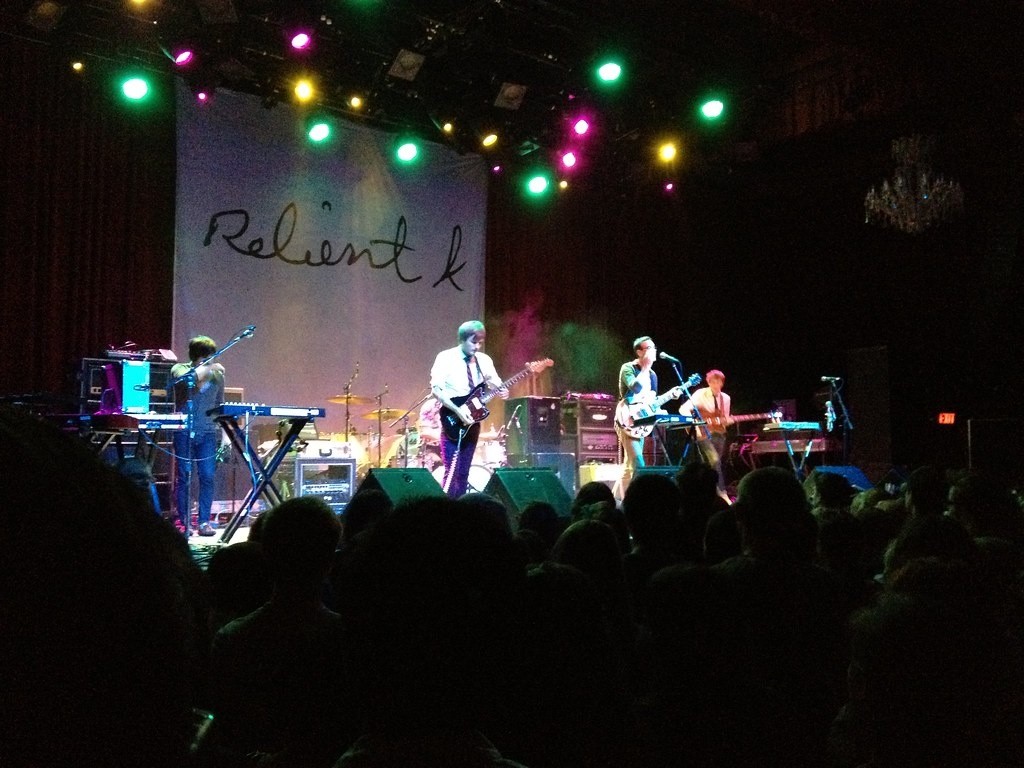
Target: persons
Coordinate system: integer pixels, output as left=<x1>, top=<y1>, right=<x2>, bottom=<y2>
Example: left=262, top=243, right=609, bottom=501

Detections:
left=678, top=370, right=731, bottom=504
left=171, top=336, right=226, bottom=535
left=431, top=320, right=509, bottom=498
left=614, top=337, right=682, bottom=497
left=0, top=408, right=1024, bottom=768
left=419, top=395, right=439, bottom=438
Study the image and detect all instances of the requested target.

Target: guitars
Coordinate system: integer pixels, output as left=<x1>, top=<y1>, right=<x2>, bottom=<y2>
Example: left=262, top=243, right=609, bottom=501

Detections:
left=686, top=406, right=783, bottom=441
left=439, top=357, right=554, bottom=441
left=614, top=373, right=702, bottom=439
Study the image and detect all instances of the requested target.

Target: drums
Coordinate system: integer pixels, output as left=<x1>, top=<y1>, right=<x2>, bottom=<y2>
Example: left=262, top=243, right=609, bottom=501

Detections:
left=419, top=398, right=443, bottom=433
left=380, top=434, right=442, bottom=468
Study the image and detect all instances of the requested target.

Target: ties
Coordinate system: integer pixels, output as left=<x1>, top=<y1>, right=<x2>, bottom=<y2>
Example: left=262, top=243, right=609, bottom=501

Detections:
left=712, top=394, right=718, bottom=412
left=465, top=359, right=474, bottom=393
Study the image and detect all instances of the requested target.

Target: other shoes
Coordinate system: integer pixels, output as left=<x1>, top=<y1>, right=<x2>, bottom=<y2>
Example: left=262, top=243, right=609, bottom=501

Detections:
left=179, top=527, right=193, bottom=535
left=198, top=523, right=216, bottom=535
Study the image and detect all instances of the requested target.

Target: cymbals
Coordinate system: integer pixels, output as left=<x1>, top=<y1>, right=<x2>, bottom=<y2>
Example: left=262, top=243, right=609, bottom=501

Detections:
left=360, top=409, right=415, bottom=420
left=478, top=432, right=505, bottom=441
left=325, top=393, right=372, bottom=405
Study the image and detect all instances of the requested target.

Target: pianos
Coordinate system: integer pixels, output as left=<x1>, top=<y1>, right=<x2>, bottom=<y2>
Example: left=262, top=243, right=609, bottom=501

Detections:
left=205, top=401, right=326, bottom=543
left=55, top=414, right=188, bottom=535
left=763, top=421, right=820, bottom=432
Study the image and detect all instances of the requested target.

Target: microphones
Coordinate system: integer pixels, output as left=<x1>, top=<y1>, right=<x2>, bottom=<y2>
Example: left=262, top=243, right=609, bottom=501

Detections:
left=507, top=407, right=518, bottom=429
left=822, top=376, right=841, bottom=382
left=244, top=326, right=256, bottom=339
left=660, top=352, right=679, bottom=363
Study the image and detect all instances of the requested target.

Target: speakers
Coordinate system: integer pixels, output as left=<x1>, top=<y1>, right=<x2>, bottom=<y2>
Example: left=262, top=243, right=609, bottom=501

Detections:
left=479, top=393, right=576, bottom=518
left=803, top=465, right=873, bottom=508
left=632, top=466, right=683, bottom=484
left=579, top=464, right=624, bottom=500
left=339, top=468, right=445, bottom=544
